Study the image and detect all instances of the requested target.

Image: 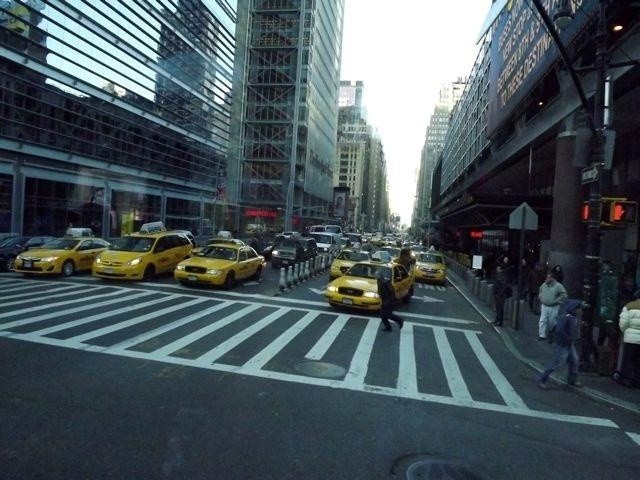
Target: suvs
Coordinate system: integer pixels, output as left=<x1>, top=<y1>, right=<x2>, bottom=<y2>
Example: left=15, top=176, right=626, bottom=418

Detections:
left=345, top=232, right=363, bottom=247
left=372, top=246, right=411, bottom=271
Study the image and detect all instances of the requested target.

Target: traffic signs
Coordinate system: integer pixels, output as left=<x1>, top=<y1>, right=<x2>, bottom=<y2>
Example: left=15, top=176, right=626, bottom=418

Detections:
left=580, top=167, right=601, bottom=184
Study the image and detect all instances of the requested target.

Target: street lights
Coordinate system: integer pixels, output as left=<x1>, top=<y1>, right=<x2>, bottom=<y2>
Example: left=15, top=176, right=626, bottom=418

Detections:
left=554, top=0, right=608, bottom=357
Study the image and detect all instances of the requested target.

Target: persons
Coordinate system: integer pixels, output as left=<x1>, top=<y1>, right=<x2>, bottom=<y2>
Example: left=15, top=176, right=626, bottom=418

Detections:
left=335, top=197, right=343, bottom=213
left=374, top=270, right=404, bottom=333
left=396, top=247, right=417, bottom=305
left=462, top=241, right=640, bottom=390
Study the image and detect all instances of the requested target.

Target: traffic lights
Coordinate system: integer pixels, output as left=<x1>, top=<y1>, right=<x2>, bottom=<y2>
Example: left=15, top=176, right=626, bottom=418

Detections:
left=611, top=201, right=637, bottom=226
left=582, top=201, right=590, bottom=222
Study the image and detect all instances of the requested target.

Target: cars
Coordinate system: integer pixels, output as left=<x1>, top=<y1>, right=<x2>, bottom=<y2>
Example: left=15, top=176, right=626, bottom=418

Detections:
left=361, top=232, right=416, bottom=249
left=174, top=243, right=267, bottom=287
left=275, top=233, right=281, bottom=238
left=414, top=245, right=450, bottom=284
left=172, top=230, right=197, bottom=248
left=0, top=235, right=58, bottom=272
left=329, top=242, right=372, bottom=280
left=14, top=227, right=119, bottom=276
left=218, top=230, right=233, bottom=237
left=326, top=250, right=414, bottom=313
left=340, top=238, right=351, bottom=250
left=238, top=233, right=258, bottom=251
left=410, top=244, right=430, bottom=261
left=191, top=238, right=245, bottom=256
left=251, top=235, right=285, bottom=257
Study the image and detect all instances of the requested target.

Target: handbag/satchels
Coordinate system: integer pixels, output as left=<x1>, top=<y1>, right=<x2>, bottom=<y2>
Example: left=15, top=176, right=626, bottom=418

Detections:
left=546, top=322, right=558, bottom=343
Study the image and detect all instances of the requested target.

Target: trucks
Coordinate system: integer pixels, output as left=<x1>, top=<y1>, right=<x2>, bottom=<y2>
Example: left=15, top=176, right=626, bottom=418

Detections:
left=308, top=232, right=341, bottom=254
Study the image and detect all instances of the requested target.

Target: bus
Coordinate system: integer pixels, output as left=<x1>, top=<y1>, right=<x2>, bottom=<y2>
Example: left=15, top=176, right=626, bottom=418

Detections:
left=310, top=225, right=343, bottom=238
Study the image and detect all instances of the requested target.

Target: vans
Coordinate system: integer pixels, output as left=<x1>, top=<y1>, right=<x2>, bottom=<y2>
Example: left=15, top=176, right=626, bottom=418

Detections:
left=282, top=231, right=299, bottom=240
left=271, top=237, right=318, bottom=268
left=91, top=220, right=193, bottom=282
